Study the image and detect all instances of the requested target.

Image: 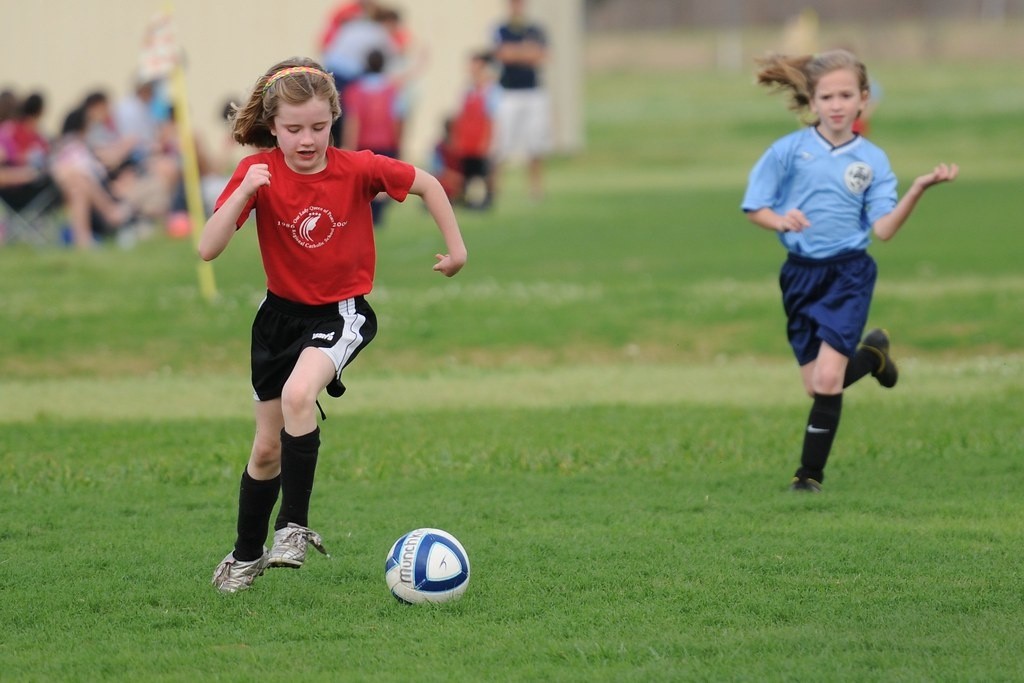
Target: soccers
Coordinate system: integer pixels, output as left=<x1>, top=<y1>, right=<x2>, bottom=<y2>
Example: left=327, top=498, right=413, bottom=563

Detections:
left=384, top=528, right=471, bottom=605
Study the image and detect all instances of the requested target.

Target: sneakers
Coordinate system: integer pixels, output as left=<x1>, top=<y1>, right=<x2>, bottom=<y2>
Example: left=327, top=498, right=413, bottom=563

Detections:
left=211, top=545, right=270, bottom=594
left=860, top=327, right=899, bottom=388
left=267, top=522, right=327, bottom=569
left=792, top=467, right=824, bottom=494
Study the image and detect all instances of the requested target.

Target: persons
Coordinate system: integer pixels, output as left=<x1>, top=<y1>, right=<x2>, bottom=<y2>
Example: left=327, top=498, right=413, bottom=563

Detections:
left=320, top=1, right=553, bottom=226
left=0, top=70, right=228, bottom=247
left=740, top=44, right=960, bottom=493
left=197, top=56, right=467, bottom=597
